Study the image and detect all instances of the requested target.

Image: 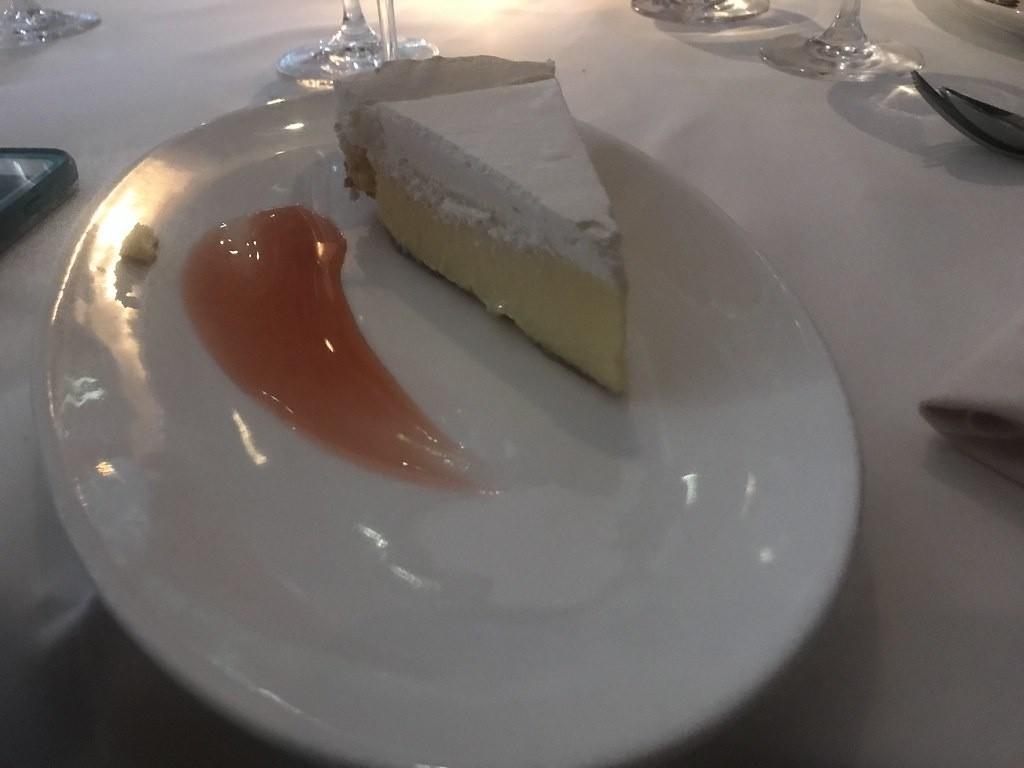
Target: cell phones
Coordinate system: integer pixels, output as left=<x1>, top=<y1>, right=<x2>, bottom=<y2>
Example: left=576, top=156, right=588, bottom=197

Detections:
left=0, top=147, right=79, bottom=254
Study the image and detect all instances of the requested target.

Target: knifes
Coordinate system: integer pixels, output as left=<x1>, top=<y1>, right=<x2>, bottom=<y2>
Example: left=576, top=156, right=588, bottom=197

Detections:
left=919, top=396, right=1024, bottom=494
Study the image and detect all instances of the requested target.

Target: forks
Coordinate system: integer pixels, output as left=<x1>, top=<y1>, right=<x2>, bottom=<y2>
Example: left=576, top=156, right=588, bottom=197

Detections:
left=910, top=71, right=1024, bottom=160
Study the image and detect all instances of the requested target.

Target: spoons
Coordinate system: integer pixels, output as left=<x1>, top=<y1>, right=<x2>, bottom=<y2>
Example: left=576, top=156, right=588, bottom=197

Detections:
left=938, top=86, right=1024, bottom=156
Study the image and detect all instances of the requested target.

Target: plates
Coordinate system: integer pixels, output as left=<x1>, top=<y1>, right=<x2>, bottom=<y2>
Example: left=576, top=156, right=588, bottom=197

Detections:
left=40, top=90, right=862, bottom=768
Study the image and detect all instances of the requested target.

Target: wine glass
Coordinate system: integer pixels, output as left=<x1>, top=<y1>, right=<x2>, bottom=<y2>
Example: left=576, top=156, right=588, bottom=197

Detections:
left=631, top=1, right=772, bottom=24
left=1, top=1, right=99, bottom=49
left=760, top=1, right=925, bottom=80
left=278, top=1, right=441, bottom=83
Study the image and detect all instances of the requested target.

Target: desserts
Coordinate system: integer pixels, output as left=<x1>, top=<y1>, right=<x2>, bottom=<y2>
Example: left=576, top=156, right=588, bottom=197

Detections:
left=331, top=51, right=630, bottom=397
